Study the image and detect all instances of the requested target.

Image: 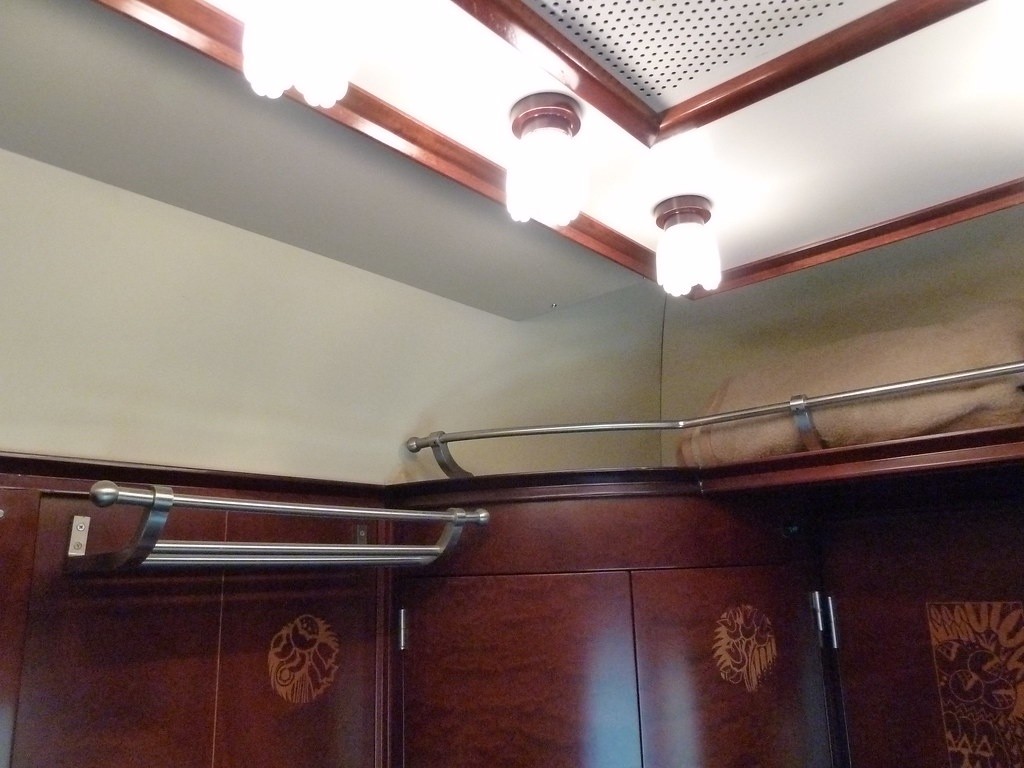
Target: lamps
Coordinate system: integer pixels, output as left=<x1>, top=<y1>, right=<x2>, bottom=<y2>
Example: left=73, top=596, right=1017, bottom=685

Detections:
left=653, top=196, right=723, bottom=296
left=505, top=94, right=581, bottom=230
